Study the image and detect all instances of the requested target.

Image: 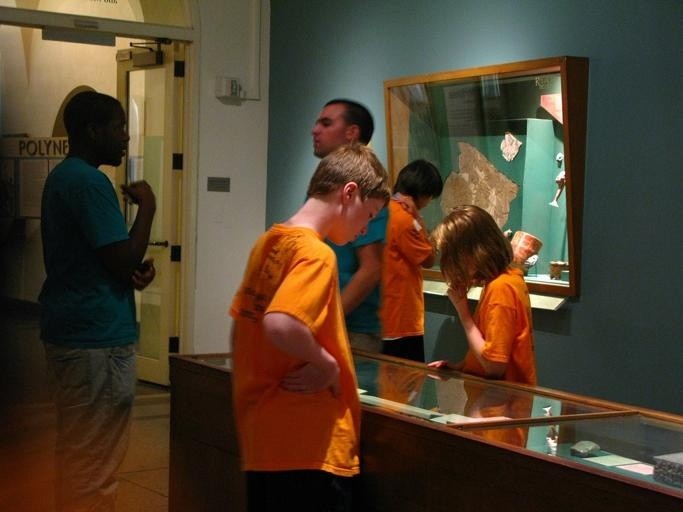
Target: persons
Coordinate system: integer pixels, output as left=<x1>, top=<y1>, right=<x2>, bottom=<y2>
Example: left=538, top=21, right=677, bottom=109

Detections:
left=386, top=160, right=444, bottom=362
left=226, top=140, right=391, bottom=512
left=427, top=203, right=539, bottom=388
left=310, top=98, right=387, bottom=358
left=425, top=371, right=534, bottom=448
left=37, top=90, right=156, bottom=512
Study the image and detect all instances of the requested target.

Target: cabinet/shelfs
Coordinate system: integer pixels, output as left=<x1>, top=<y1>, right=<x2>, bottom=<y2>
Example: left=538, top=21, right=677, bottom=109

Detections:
left=165, top=341, right=682, bottom=511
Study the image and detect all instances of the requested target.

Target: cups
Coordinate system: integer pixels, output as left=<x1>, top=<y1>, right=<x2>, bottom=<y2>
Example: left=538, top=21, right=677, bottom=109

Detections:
left=508, top=230, right=542, bottom=274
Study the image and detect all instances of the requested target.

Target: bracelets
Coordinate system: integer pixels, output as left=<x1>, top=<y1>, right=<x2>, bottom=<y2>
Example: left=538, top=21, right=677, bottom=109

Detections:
left=415, top=212, right=423, bottom=223
